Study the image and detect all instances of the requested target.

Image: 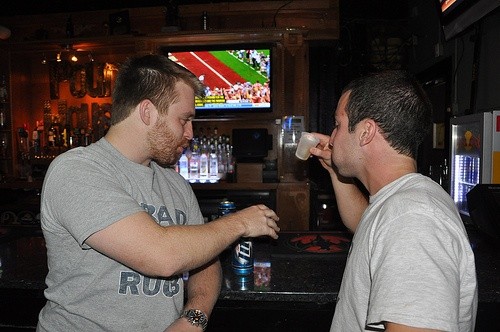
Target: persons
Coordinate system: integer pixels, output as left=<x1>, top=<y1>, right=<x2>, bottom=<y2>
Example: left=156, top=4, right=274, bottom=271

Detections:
left=307, top=70, right=478, bottom=331
left=36, top=53, right=280, bottom=331
left=199, top=49, right=270, bottom=102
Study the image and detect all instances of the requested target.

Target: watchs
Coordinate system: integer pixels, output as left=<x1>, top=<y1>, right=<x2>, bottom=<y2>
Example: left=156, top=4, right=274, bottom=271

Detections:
left=179, top=308, right=208, bottom=329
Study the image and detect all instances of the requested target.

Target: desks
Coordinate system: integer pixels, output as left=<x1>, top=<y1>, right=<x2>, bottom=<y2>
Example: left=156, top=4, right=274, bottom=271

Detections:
left=0, top=233, right=351, bottom=332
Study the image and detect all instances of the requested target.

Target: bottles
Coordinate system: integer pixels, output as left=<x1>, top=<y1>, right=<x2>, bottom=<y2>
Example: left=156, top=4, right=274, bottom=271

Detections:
left=173, top=127, right=233, bottom=182
left=18, top=119, right=110, bottom=156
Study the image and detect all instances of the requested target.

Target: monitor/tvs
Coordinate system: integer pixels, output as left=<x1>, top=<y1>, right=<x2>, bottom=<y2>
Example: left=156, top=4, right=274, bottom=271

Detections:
left=163, top=41, right=273, bottom=117
left=232, top=126, right=268, bottom=162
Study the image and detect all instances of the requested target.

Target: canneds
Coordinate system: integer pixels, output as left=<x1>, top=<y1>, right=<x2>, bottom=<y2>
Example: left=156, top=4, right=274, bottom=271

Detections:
left=233, top=237, right=253, bottom=276
left=233, top=268, right=253, bottom=290
left=218, top=201, right=236, bottom=218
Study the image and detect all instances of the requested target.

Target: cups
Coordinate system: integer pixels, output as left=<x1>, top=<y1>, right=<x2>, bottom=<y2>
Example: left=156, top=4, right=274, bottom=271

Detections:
left=295, top=132, right=320, bottom=160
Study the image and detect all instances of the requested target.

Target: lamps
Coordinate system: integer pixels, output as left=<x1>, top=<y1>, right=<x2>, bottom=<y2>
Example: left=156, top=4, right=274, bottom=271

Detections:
left=40, top=44, right=94, bottom=64
